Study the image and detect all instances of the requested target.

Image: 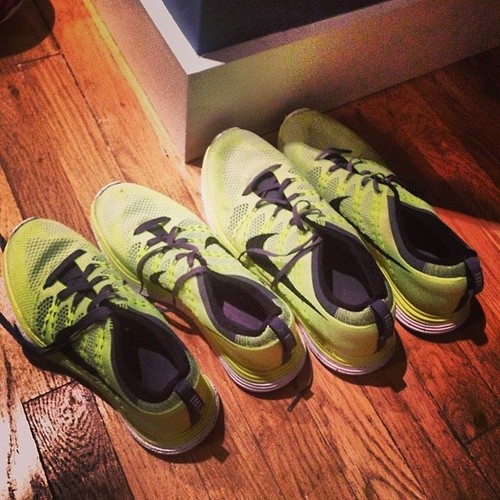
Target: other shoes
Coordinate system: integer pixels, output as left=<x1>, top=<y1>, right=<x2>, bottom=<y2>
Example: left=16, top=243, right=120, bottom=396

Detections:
left=0, top=1, right=23, bottom=26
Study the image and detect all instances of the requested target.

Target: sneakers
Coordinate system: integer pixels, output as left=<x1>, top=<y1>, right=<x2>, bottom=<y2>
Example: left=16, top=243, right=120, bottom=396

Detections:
left=3, top=218, right=220, bottom=455
left=278, top=107, right=483, bottom=338
left=91, top=181, right=307, bottom=394
left=198, top=126, right=398, bottom=377
